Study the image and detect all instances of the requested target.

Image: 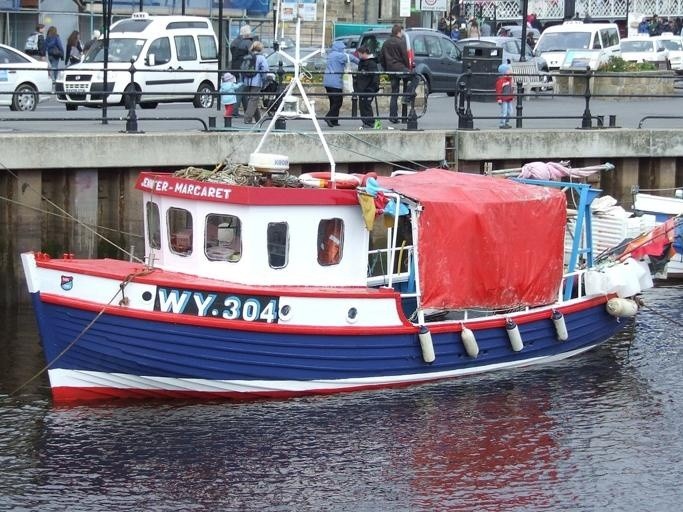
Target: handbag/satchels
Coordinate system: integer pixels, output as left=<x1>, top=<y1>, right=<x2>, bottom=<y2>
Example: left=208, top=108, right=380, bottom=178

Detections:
left=68, top=45, right=80, bottom=61
left=48, top=43, right=64, bottom=59
left=341, top=52, right=354, bottom=96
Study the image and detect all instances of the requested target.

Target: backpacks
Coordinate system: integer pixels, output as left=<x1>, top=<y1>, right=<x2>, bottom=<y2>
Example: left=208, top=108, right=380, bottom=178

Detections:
left=24, top=32, right=44, bottom=52
left=240, top=52, right=257, bottom=78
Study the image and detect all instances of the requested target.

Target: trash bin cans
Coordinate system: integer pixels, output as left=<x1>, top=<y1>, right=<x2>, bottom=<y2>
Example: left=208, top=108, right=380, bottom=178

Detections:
left=261, top=80, right=277, bottom=107
left=462, top=46, right=503, bottom=103
left=510, top=63, right=539, bottom=82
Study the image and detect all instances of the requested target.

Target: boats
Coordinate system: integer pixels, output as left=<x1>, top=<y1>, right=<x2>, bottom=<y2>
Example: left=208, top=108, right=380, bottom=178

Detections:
left=23, top=167, right=646, bottom=412
left=631, top=188, right=683, bottom=288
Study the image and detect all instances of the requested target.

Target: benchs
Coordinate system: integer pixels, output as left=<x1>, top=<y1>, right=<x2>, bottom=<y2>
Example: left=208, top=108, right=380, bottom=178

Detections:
left=510, top=62, right=557, bottom=101
left=665, top=56, right=683, bottom=89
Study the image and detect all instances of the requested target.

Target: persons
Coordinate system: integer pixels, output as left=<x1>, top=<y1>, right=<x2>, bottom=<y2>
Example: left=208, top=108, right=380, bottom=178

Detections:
left=499, top=28, right=513, bottom=37
left=381, top=24, right=419, bottom=122
left=639, top=14, right=683, bottom=37
left=526, top=32, right=535, bottom=49
left=527, top=14, right=535, bottom=28
left=355, top=46, right=379, bottom=129
left=496, top=62, right=515, bottom=130
left=322, top=40, right=349, bottom=128
left=24, top=23, right=101, bottom=82
left=438, top=14, right=492, bottom=40
left=221, top=23, right=270, bottom=123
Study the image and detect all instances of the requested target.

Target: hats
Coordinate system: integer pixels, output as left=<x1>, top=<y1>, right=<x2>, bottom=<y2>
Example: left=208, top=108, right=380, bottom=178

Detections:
left=222, top=71, right=236, bottom=82
left=497, top=63, right=511, bottom=72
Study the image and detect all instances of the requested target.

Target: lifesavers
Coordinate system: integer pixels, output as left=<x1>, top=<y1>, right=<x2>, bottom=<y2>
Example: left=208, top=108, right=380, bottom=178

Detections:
left=299, top=172, right=358, bottom=189
